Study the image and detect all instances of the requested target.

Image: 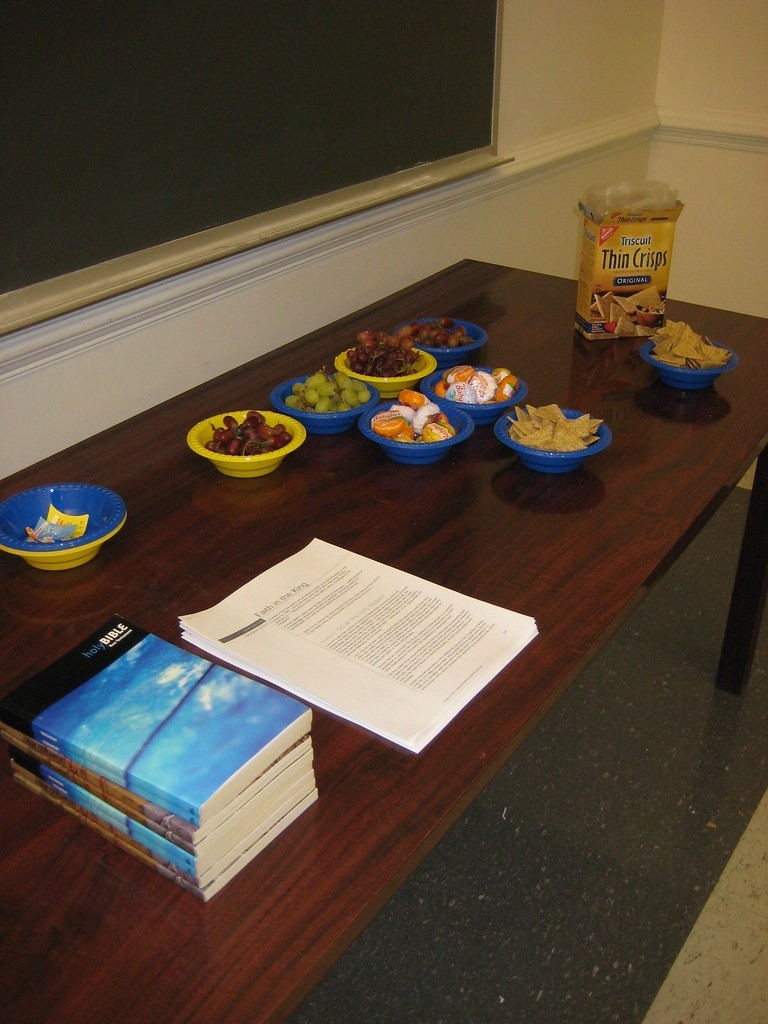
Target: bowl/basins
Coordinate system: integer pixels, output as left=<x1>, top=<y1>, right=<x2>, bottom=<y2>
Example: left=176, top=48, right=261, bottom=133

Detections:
left=0, top=482, right=126, bottom=551
left=188, top=411, right=306, bottom=477
left=419, top=367, right=528, bottom=423
left=638, top=340, right=739, bottom=389
left=493, top=409, right=613, bottom=473
left=270, top=376, right=380, bottom=433
left=334, top=347, right=437, bottom=395
left=359, top=403, right=476, bottom=464
left=0, top=514, right=126, bottom=571
left=392, top=318, right=488, bottom=367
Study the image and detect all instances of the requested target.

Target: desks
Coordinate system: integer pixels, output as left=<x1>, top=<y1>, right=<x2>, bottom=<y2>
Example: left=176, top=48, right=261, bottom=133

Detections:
left=0, top=254, right=768, bottom=1023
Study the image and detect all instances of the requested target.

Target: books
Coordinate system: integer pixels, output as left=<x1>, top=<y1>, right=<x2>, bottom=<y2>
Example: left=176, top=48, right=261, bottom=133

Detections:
left=0, top=613, right=318, bottom=904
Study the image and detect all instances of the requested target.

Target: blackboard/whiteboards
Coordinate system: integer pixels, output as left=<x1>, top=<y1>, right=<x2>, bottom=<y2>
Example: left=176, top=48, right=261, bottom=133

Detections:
left=0, top=0, right=516, bottom=339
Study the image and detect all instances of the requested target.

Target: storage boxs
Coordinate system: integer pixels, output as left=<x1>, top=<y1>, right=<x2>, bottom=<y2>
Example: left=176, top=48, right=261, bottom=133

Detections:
left=574, top=197, right=683, bottom=340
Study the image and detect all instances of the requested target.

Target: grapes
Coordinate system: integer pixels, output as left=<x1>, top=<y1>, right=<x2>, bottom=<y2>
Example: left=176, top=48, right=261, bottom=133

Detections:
left=285, top=372, right=370, bottom=412
left=205, top=413, right=291, bottom=454
left=398, top=318, right=476, bottom=348
left=346, top=329, right=426, bottom=376
